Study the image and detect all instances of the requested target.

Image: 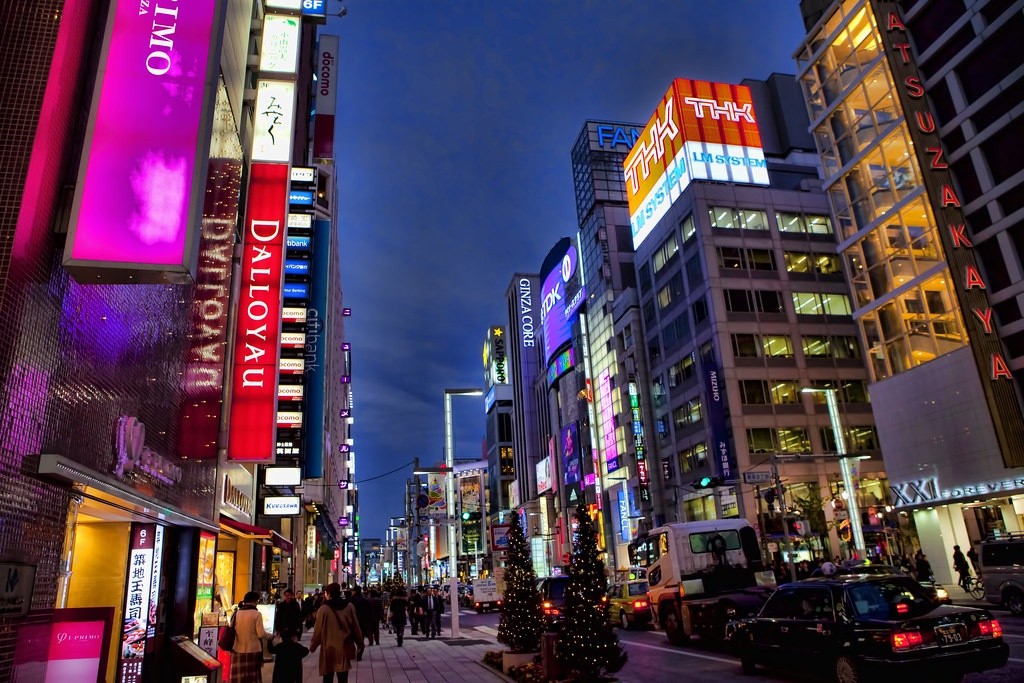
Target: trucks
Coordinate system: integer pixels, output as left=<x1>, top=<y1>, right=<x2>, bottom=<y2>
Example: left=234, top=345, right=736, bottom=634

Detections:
left=627, top=519, right=760, bottom=631
left=472, top=578, right=506, bottom=615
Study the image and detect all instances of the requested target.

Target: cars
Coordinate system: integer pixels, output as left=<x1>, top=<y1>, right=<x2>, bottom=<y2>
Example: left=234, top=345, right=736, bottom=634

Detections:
left=733, top=573, right=1010, bottom=683
left=605, top=579, right=650, bottom=632
left=812, top=563, right=954, bottom=607
left=698, top=586, right=777, bottom=656
left=436, top=582, right=472, bottom=607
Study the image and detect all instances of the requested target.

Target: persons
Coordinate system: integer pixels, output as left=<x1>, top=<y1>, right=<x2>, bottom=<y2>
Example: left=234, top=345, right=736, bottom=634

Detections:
left=230, top=582, right=364, bottom=683
left=296, top=581, right=373, bottom=661
left=374, top=581, right=402, bottom=630
left=390, top=589, right=411, bottom=647
left=407, top=585, right=445, bottom=638
left=1007, top=532, right=1013, bottom=538
left=966, top=546, right=982, bottom=587
left=770, top=555, right=902, bottom=572
left=953, top=546, right=971, bottom=592
left=908, top=548, right=935, bottom=585
left=366, top=590, right=385, bottom=646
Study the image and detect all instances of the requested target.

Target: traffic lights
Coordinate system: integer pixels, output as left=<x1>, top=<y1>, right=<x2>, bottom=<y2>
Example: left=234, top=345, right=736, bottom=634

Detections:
left=461, top=512, right=483, bottom=521
left=695, top=476, right=720, bottom=490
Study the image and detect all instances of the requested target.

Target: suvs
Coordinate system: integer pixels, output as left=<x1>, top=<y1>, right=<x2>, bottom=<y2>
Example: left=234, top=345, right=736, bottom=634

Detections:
left=978, top=532, right=1024, bottom=613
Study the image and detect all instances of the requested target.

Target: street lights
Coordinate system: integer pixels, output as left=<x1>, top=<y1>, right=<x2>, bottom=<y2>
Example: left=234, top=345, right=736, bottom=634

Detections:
left=443, top=386, right=485, bottom=638
left=533, top=525, right=561, bottom=576
left=770, top=452, right=874, bottom=580
left=800, top=387, right=868, bottom=561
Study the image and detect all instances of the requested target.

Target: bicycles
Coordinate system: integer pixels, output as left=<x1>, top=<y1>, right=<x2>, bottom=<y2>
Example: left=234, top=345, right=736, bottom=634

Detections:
left=964, top=573, right=985, bottom=600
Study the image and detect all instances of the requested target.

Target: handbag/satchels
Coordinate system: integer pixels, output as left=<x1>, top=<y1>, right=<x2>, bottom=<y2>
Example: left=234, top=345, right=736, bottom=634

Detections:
left=414, top=607, right=424, bottom=616
left=217, top=612, right=237, bottom=653
left=386, top=605, right=393, bottom=625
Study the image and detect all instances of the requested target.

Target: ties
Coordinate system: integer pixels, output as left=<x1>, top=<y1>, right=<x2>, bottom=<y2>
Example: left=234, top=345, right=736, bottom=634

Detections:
left=429, top=596, right=431, bottom=610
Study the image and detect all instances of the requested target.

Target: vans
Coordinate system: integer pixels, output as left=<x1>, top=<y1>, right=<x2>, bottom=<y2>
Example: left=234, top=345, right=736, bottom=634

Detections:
left=535, top=576, right=574, bottom=629
